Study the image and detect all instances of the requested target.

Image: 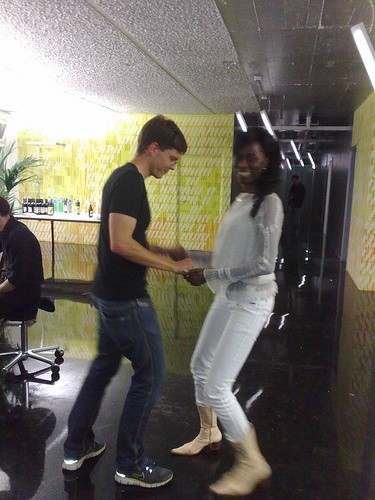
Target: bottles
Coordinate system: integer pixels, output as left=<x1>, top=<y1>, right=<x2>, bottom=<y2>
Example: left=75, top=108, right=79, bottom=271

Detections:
left=22, top=197, right=93, bottom=218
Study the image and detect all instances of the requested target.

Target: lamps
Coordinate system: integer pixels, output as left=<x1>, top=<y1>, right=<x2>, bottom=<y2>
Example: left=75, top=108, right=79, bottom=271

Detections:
left=349, top=0, right=375, bottom=90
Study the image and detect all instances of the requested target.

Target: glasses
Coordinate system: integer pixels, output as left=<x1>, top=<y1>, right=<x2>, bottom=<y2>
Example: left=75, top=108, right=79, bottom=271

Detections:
left=164, top=147, right=178, bottom=167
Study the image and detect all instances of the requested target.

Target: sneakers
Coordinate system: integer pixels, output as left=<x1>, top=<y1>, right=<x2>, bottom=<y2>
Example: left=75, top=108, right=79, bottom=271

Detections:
left=62, top=439, right=106, bottom=471
left=114, top=457, right=172, bottom=488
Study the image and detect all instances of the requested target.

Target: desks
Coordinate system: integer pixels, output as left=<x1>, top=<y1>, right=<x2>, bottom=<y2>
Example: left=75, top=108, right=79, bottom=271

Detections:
left=14, top=211, right=101, bottom=285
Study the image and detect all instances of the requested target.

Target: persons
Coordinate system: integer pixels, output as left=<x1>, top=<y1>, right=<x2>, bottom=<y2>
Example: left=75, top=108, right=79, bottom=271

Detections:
left=171, top=130, right=284, bottom=495
left=61, top=113, right=194, bottom=488
left=0, top=196, right=43, bottom=389
left=286, top=174, right=305, bottom=238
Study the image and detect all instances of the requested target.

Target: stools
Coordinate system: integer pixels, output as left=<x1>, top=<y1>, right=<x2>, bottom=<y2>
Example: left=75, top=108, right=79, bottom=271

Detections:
left=0, top=293, right=66, bottom=380
left=0, top=357, right=64, bottom=442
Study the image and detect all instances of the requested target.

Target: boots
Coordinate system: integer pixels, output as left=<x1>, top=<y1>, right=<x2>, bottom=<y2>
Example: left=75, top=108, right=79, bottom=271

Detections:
left=208, top=422, right=272, bottom=500
left=170, top=407, right=222, bottom=456
